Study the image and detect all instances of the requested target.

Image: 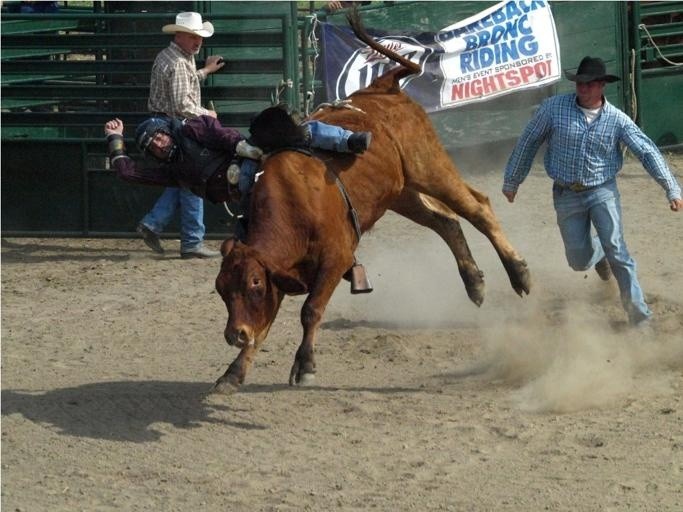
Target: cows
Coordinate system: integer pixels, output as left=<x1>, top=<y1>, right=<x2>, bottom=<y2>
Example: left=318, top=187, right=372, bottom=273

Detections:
left=209, top=6, right=532, bottom=395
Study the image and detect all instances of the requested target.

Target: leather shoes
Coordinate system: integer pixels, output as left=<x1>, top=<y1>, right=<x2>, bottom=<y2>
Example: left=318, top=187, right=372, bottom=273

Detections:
left=594, top=257, right=612, bottom=282
left=137, top=223, right=165, bottom=254
left=179, top=244, right=222, bottom=260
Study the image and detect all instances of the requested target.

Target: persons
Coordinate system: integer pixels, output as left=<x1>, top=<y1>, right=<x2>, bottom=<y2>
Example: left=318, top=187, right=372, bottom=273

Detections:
left=502, top=58, right=681, bottom=327
left=104, top=114, right=373, bottom=253
left=135, top=11, right=227, bottom=261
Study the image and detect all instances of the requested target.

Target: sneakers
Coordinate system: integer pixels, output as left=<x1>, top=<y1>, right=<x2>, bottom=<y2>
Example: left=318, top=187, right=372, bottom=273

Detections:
left=347, top=129, right=374, bottom=154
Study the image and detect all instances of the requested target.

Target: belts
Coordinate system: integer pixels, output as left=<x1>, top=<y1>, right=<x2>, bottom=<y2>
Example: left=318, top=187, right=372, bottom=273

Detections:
left=552, top=176, right=617, bottom=193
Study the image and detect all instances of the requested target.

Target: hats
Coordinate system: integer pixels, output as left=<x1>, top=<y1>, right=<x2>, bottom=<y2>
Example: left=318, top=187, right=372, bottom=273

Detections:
left=563, top=55, right=622, bottom=85
left=161, top=11, right=215, bottom=39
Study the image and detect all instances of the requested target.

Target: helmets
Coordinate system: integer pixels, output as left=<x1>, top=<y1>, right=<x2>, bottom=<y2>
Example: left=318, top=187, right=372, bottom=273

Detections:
left=133, top=116, right=178, bottom=165
